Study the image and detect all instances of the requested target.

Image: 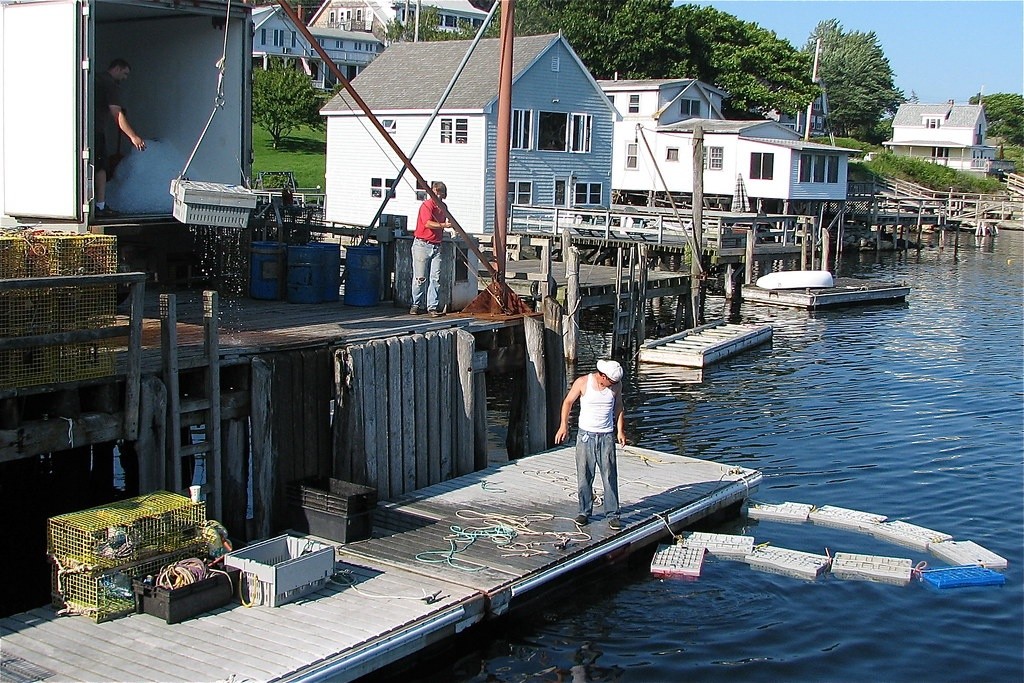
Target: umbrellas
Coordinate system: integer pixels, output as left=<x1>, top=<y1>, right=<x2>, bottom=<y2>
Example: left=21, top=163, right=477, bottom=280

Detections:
left=732, top=173, right=750, bottom=212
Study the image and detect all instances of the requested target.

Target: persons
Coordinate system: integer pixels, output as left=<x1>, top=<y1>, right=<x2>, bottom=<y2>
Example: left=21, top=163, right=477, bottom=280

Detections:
left=94, top=59, right=146, bottom=217
left=410, top=181, right=452, bottom=317
left=555, top=360, right=626, bottom=531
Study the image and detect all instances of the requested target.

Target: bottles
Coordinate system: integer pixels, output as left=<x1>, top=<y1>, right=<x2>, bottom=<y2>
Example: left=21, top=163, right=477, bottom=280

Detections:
left=121, top=258, right=130, bottom=287
left=395, top=218, right=401, bottom=237
left=117, top=261, right=122, bottom=288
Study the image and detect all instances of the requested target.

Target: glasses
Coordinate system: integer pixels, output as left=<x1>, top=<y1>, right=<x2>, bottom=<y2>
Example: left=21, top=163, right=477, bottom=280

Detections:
left=603, top=376, right=620, bottom=385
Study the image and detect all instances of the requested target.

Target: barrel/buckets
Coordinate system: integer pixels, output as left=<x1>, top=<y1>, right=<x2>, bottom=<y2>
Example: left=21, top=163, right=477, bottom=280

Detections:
left=307, top=243, right=340, bottom=301
left=286, top=246, right=322, bottom=304
left=344, top=246, right=381, bottom=306
left=252, top=241, right=286, bottom=300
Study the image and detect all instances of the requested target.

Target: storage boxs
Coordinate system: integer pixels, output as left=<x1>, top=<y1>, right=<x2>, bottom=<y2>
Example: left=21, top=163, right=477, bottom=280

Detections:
left=0, top=178, right=260, bottom=395
left=650, top=501, right=1009, bottom=590
left=46, top=478, right=416, bottom=624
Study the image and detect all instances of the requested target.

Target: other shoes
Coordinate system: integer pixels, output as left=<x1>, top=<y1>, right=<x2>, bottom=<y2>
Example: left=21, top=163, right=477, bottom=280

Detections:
left=410, top=307, right=420, bottom=315
left=608, top=518, right=622, bottom=530
left=428, top=309, right=438, bottom=318
left=572, top=515, right=588, bottom=526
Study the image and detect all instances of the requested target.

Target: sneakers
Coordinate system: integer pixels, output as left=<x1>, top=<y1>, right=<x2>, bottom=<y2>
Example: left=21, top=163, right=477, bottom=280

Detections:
left=95, top=202, right=121, bottom=218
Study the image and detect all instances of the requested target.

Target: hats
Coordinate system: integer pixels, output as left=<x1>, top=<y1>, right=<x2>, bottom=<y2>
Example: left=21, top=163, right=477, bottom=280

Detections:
left=596, top=359, right=623, bottom=382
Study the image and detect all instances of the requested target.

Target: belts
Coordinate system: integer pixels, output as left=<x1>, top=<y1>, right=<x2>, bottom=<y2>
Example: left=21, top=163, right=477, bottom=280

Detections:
left=416, top=237, right=440, bottom=246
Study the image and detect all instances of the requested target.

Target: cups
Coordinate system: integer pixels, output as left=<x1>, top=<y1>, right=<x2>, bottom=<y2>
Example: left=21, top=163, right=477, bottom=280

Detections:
left=190, top=485, right=201, bottom=502
